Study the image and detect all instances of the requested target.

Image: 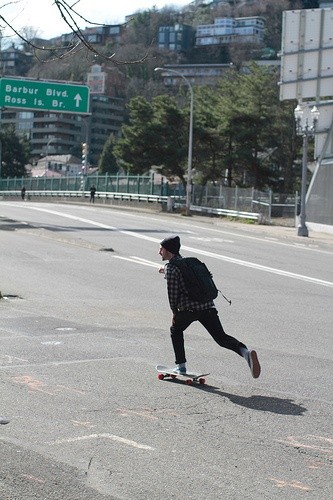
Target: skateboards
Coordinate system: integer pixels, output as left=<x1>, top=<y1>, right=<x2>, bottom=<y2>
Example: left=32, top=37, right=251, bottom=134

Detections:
left=153, top=363, right=211, bottom=387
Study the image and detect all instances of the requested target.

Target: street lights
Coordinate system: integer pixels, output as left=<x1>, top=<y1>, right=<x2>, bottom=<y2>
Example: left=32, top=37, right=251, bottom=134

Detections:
left=155, top=67, right=195, bottom=216
left=293, top=105, right=320, bottom=238
left=43, top=138, right=61, bottom=189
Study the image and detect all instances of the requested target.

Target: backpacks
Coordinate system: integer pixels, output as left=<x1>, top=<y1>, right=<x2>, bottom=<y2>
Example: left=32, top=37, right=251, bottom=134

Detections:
left=170, top=257, right=218, bottom=302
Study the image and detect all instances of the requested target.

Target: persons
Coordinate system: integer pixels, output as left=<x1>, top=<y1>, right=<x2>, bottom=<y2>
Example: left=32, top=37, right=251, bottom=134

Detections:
left=20, top=186, right=26, bottom=199
left=88, top=184, right=96, bottom=203
left=159, top=235, right=261, bottom=378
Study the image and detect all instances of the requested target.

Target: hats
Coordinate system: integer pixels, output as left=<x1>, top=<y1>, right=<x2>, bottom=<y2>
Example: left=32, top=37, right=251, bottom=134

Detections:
left=160, top=236, right=181, bottom=254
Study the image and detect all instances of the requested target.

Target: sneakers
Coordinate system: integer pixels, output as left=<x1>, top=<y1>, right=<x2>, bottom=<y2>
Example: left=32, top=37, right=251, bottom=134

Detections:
left=173, top=367, right=186, bottom=374
left=247, top=350, right=261, bottom=378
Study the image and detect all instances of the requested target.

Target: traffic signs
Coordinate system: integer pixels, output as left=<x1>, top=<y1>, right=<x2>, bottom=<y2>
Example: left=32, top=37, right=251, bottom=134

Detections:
left=0, top=75, right=91, bottom=116
